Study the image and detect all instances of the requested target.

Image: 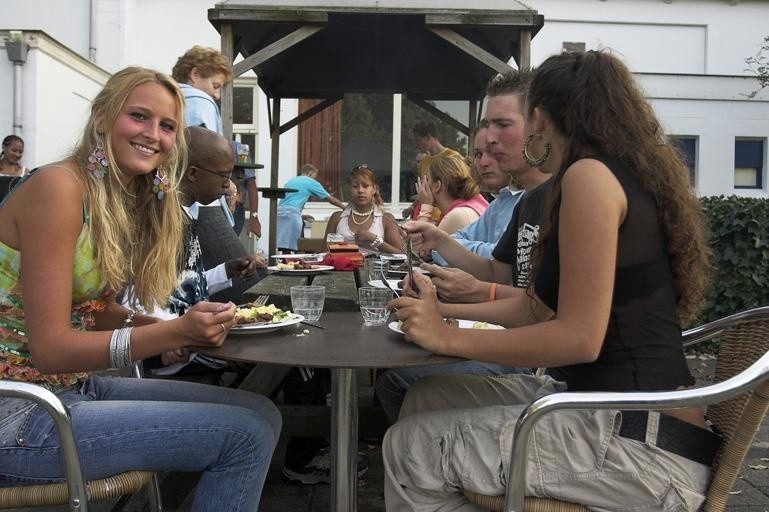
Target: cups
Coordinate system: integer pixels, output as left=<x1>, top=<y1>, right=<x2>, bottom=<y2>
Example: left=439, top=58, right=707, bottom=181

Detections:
left=359, top=286, right=393, bottom=324
left=290, top=286, right=326, bottom=323
left=237, top=144, right=248, bottom=163
left=365, top=257, right=389, bottom=285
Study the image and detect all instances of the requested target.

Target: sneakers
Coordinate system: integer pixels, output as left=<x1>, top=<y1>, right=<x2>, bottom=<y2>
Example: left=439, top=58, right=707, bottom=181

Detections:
left=281, top=448, right=370, bottom=485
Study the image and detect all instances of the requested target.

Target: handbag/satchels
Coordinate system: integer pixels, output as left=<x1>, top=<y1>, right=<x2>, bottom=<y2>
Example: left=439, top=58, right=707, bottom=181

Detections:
left=327, top=243, right=365, bottom=267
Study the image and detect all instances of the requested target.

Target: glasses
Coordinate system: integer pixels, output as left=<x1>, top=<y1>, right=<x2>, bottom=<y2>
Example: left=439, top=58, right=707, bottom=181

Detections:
left=196, top=165, right=232, bottom=181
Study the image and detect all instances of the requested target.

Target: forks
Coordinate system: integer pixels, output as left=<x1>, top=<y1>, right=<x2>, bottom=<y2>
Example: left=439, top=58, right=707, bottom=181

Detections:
left=214, top=294, right=269, bottom=310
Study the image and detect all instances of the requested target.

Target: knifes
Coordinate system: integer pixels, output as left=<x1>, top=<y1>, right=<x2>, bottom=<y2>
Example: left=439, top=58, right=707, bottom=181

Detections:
left=406, top=235, right=414, bottom=289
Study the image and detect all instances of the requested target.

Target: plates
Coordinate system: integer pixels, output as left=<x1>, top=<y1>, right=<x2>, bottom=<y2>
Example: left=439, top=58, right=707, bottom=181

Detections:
left=367, top=280, right=405, bottom=290
left=223, top=312, right=304, bottom=335
left=266, top=252, right=335, bottom=276
left=389, top=318, right=506, bottom=336
left=380, top=254, right=407, bottom=263
left=387, top=265, right=440, bottom=274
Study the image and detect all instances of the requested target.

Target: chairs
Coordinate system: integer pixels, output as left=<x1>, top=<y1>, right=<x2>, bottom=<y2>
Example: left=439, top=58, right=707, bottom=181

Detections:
left=462, top=303, right=769, bottom=507
left=0, top=369, right=163, bottom=507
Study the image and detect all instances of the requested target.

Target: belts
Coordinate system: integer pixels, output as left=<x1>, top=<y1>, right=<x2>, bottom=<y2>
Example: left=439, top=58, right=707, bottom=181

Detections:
left=620, top=410, right=724, bottom=467
